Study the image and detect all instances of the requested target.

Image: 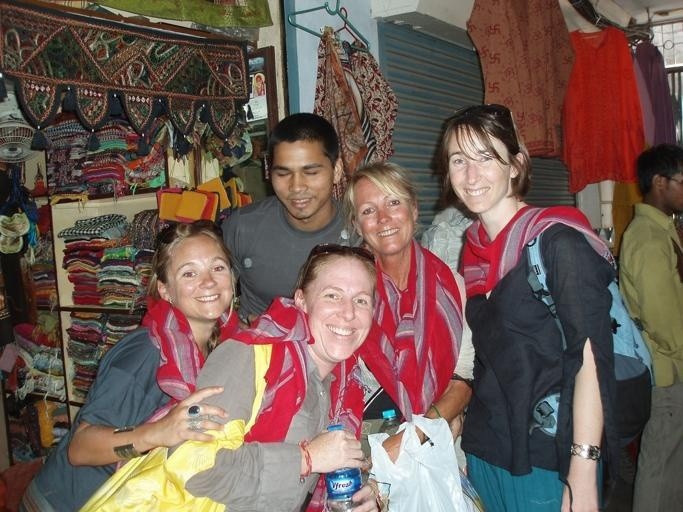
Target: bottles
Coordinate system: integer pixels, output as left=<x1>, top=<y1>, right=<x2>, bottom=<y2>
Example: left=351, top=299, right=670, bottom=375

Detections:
left=377, top=409, right=401, bottom=439
left=325, top=425, right=365, bottom=511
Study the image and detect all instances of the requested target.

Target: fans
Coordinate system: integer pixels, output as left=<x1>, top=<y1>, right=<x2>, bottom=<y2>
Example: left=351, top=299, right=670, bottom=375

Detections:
left=0, top=114, right=40, bottom=165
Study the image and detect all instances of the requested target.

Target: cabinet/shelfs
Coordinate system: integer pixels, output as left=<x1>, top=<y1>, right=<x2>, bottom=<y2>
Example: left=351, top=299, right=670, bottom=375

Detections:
left=0, top=149, right=197, bottom=469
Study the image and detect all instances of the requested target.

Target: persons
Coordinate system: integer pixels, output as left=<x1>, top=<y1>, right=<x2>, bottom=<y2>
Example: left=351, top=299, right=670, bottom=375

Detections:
left=344, top=160, right=465, bottom=480
left=218, top=111, right=365, bottom=333
left=80, top=243, right=384, bottom=512
left=17, top=218, right=238, bottom=511
left=421, top=104, right=640, bottom=512
left=619, top=143, right=683, bottom=511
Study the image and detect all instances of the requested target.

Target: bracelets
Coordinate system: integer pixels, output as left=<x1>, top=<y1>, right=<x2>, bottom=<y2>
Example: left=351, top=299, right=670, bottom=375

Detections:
left=297, top=441, right=314, bottom=482
left=113, top=426, right=152, bottom=461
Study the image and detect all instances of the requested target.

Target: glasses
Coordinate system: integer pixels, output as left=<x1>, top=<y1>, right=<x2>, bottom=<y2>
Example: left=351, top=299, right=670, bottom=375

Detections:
left=161, top=219, right=216, bottom=243
left=313, top=242, right=374, bottom=262
left=463, top=103, right=521, bottom=147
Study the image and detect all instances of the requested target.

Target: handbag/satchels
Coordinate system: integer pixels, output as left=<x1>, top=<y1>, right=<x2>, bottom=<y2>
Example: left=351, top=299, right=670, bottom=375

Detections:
left=81, top=446, right=231, bottom=512
left=527, top=209, right=654, bottom=446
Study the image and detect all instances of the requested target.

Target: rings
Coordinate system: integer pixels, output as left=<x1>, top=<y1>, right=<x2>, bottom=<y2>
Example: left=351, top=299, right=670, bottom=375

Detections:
left=187, top=418, right=207, bottom=432
left=186, top=404, right=203, bottom=419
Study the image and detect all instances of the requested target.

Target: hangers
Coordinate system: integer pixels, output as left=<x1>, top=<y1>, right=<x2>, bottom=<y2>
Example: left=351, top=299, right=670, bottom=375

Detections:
left=288, top=0, right=371, bottom=54
left=578, top=13, right=606, bottom=32
left=622, top=26, right=649, bottom=40
